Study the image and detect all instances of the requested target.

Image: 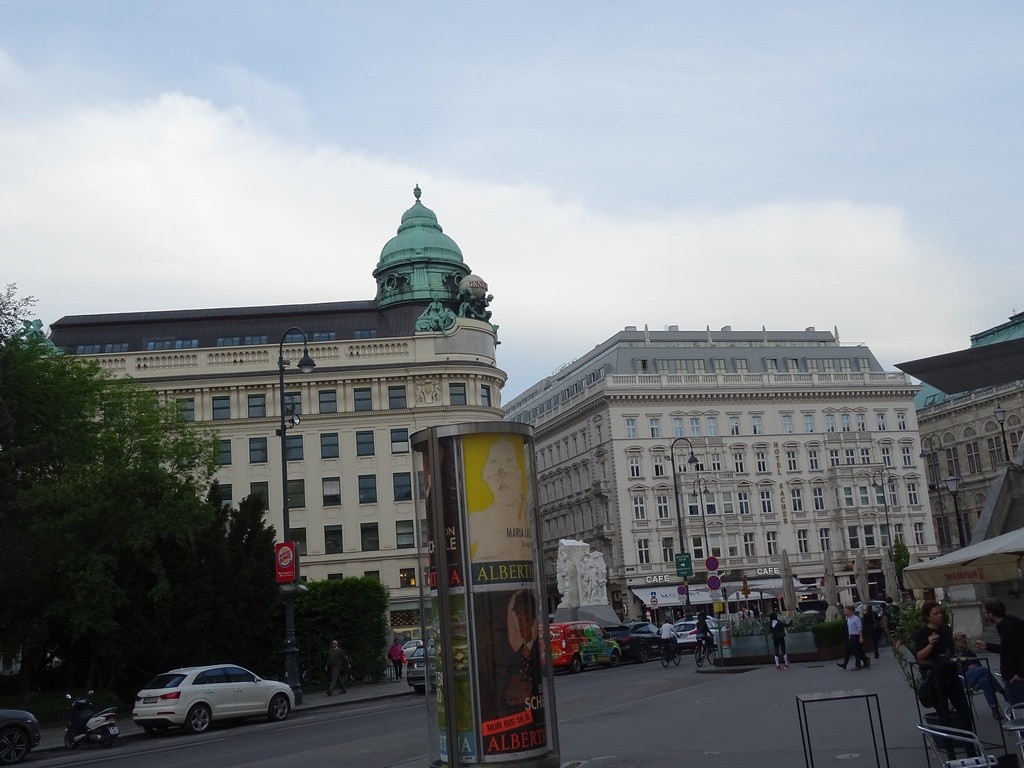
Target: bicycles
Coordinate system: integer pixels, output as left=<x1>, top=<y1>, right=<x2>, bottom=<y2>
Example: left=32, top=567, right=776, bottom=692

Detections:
left=694, top=637, right=716, bottom=667
left=661, top=633, right=682, bottom=668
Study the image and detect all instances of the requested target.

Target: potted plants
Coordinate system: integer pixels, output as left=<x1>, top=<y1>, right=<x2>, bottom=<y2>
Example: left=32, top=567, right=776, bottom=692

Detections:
left=881, top=589, right=971, bottom=748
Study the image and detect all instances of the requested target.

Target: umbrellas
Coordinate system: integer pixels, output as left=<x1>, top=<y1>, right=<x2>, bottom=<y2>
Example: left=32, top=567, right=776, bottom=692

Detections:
left=902, top=526, right=1024, bottom=589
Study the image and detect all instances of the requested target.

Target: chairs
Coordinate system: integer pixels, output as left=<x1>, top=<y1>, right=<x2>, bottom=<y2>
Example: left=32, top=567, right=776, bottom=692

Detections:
left=916, top=675, right=1024, bottom=768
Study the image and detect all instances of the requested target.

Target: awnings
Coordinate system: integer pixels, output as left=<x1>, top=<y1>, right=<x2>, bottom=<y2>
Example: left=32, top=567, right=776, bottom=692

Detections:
left=631, top=577, right=811, bottom=607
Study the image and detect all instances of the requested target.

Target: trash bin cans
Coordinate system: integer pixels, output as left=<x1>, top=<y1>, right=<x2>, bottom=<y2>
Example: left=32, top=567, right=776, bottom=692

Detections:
left=718, top=620, right=731, bottom=649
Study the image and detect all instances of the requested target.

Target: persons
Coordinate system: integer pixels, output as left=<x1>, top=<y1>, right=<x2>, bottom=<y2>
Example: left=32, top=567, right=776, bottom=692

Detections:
left=769, top=613, right=793, bottom=671
left=696, top=618, right=715, bottom=662
left=388, top=639, right=404, bottom=680
left=904, top=595, right=913, bottom=603
left=836, top=597, right=901, bottom=671
left=972, top=598, right=1024, bottom=710
left=914, top=601, right=979, bottom=760
left=325, top=640, right=352, bottom=696
left=952, top=631, right=1009, bottom=720
left=659, top=619, right=680, bottom=661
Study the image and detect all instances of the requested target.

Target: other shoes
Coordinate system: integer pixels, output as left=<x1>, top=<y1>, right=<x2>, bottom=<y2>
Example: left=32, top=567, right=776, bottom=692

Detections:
left=396, top=676, right=398, bottom=679
left=776, top=665, right=780, bottom=671
left=784, top=664, right=790, bottom=670
left=698, top=656, right=701, bottom=662
left=326, top=692, right=331, bottom=696
left=851, top=665, right=862, bottom=670
left=862, top=664, right=870, bottom=668
left=707, top=648, right=710, bottom=654
left=875, top=654, right=880, bottom=658
left=339, top=691, right=346, bottom=694
left=836, top=663, right=846, bottom=669
left=399, top=675, right=402, bottom=678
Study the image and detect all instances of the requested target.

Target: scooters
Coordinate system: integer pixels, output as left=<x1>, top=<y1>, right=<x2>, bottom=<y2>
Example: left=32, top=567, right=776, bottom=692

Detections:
left=62, top=690, right=120, bottom=748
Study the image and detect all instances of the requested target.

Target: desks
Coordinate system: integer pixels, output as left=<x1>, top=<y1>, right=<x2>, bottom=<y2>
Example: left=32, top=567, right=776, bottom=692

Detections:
left=909, top=657, right=1009, bottom=768
left=795, top=689, right=892, bottom=768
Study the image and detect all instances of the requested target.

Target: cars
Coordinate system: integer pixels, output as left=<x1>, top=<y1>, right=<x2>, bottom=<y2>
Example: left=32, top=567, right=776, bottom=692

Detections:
left=850, top=600, right=888, bottom=617
left=401, top=639, right=423, bottom=661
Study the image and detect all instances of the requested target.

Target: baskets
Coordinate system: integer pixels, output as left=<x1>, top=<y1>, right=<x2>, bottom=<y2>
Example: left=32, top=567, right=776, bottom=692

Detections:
left=707, top=636, right=713, bottom=645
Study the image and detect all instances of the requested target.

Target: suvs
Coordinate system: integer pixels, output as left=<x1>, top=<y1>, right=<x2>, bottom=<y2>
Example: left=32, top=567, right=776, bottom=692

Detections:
left=407, top=645, right=425, bottom=693
left=0, top=710, right=42, bottom=763
left=132, top=662, right=296, bottom=734
left=671, top=619, right=719, bottom=650
left=600, top=622, right=661, bottom=664
left=538, top=620, right=622, bottom=673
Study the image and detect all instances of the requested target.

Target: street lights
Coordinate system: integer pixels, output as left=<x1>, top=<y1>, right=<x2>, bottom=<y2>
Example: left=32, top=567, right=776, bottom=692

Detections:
left=670, top=437, right=699, bottom=620
left=943, top=472, right=962, bottom=549
left=918, top=434, right=953, bottom=552
left=691, top=477, right=710, bottom=559
left=993, top=403, right=1010, bottom=461
left=871, top=468, right=895, bottom=561
left=275, top=325, right=318, bottom=709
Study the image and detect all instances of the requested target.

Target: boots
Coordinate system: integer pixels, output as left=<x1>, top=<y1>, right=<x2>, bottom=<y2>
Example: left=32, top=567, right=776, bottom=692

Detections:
left=999, top=686, right=1011, bottom=702
left=991, top=706, right=1003, bottom=720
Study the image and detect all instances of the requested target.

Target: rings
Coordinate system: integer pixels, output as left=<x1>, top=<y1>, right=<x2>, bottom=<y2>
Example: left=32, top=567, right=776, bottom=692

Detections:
left=932, top=637, right=933, bottom=640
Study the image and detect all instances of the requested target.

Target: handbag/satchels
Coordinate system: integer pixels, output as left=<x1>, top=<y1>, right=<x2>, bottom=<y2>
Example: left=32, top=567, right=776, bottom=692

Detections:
left=401, top=654, right=406, bottom=663
left=873, top=623, right=881, bottom=631
left=918, top=681, right=937, bottom=708
